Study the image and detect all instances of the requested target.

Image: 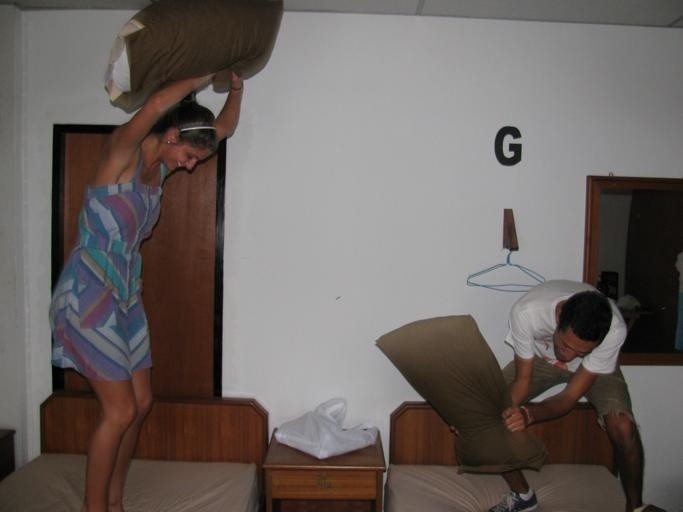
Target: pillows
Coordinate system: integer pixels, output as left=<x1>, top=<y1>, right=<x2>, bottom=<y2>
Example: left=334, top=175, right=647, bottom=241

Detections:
left=100, top=0, right=285, bottom=116
left=372, top=313, right=552, bottom=477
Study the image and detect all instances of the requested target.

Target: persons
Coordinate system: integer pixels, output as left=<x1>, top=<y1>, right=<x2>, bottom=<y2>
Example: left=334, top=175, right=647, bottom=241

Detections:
left=487, top=278, right=665, bottom=511
left=48, top=59, right=247, bottom=511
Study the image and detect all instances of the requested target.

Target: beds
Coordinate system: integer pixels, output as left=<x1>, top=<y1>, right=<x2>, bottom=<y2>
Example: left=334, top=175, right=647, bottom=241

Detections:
left=0, top=389, right=270, bottom=511
left=381, top=398, right=629, bottom=512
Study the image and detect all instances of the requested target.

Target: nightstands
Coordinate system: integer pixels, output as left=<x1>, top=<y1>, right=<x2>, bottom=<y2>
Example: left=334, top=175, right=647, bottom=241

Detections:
left=260, top=425, right=387, bottom=511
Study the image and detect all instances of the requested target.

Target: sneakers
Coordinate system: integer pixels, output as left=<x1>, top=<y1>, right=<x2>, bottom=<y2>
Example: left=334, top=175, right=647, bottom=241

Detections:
left=485, top=489, right=539, bottom=512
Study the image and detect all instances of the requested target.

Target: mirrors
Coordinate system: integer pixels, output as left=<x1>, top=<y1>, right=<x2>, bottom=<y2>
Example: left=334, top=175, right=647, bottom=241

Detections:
left=582, top=175, right=683, bottom=367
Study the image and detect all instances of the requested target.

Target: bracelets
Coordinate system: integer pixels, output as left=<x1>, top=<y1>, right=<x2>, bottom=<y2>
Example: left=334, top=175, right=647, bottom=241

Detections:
left=228, top=86, right=241, bottom=93
left=519, top=405, right=532, bottom=425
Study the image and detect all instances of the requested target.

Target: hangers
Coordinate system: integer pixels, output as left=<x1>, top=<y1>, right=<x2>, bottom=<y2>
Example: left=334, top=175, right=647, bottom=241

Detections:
left=464, top=244, right=546, bottom=294
